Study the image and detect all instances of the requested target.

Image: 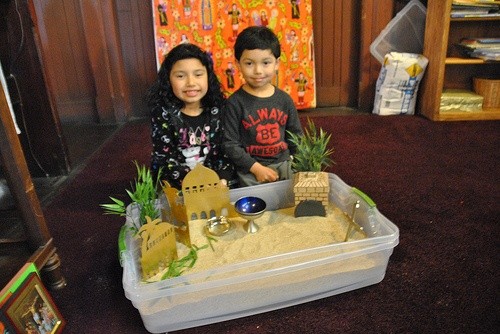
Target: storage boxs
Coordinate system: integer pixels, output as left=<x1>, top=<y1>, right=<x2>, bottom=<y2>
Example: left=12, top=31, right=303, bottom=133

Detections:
left=117, top=171, right=400, bottom=334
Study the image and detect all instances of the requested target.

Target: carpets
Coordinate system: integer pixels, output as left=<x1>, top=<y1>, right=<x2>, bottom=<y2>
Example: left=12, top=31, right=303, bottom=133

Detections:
left=21, top=114, right=500, bottom=334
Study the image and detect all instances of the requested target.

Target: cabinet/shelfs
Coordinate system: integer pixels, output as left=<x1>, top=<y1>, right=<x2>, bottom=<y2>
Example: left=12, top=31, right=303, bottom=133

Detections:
left=416, top=0, right=500, bottom=122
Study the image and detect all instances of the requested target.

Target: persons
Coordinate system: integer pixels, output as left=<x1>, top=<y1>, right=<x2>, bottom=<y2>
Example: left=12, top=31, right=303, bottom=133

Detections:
left=21, top=295, right=56, bottom=334
left=146, top=44, right=236, bottom=194
left=221, top=26, right=304, bottom=187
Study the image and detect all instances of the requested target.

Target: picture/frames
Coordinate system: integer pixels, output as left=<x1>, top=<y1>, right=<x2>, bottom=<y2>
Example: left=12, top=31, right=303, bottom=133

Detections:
left=0, top=272, right=68, bottom=334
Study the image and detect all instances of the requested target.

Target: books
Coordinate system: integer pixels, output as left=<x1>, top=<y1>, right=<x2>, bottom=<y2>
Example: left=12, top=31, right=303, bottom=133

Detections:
left=451, top=0, right=500, bottom=18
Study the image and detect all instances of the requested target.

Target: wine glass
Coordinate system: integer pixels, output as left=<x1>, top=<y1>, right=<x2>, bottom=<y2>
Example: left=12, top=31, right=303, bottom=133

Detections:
left=234, top=196, right=267, bottom=233
